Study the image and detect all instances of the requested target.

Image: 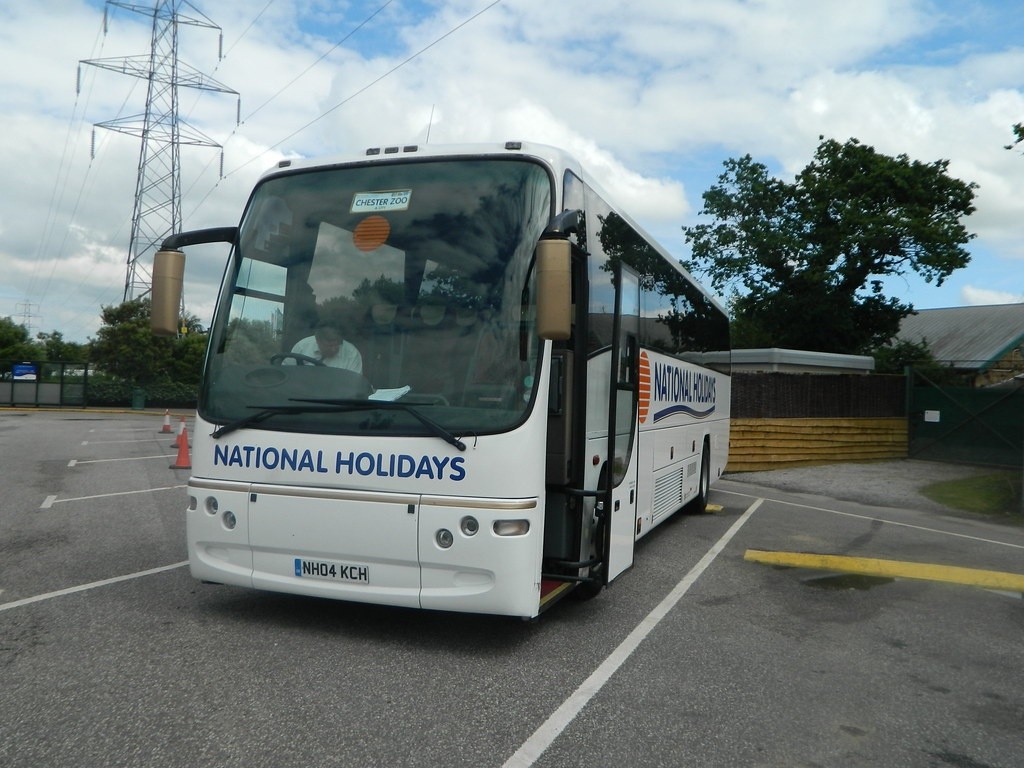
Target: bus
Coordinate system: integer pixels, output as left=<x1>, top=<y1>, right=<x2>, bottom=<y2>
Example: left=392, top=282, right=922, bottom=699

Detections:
left=149, top=139, right=735, bottom=624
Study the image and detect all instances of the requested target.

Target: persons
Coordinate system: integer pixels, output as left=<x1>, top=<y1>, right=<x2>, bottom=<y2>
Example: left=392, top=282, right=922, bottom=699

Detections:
left=280, top=318, right=363, bottom=378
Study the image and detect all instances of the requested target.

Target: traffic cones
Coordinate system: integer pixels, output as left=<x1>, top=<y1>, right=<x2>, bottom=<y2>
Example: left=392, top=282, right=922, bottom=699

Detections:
left=168, top=426, right=192, bottom=470
left=169, top=415, right=193, bottom=450
left=157, top=408, right=175, bottom=434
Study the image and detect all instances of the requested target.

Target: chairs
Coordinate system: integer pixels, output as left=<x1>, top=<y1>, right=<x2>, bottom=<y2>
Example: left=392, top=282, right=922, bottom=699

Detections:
left=344, top=298, right=575, bottom=412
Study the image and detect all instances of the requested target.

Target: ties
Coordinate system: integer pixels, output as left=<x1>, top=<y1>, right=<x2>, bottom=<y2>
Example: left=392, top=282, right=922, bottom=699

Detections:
left=317, top=355, right=328, bottom=363
